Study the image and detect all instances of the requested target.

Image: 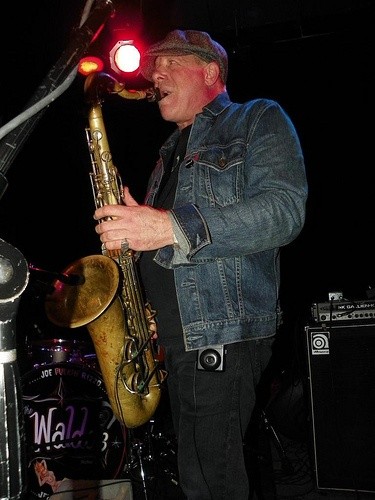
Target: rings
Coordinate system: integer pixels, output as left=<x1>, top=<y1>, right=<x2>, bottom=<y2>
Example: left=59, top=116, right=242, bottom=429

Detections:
left=121, top=239, right=129, bottom=251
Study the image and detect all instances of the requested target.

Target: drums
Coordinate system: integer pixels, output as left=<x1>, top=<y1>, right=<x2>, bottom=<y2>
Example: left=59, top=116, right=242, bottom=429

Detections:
left=17, top=361, right=128, bottom=497
left=41, top=338, right=89, bottom=366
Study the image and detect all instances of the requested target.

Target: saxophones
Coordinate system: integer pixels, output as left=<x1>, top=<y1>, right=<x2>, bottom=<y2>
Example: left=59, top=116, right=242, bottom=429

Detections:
left=46, top=70, right=162, bottom=428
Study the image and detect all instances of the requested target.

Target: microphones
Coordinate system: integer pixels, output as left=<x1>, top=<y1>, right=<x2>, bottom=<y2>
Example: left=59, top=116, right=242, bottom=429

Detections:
left=29, top=266, right=86, bottom=286
left=75, top=0, right=115, bottom=51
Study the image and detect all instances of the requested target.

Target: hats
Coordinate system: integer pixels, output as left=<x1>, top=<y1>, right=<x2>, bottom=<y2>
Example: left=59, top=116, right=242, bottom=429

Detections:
left=140, top=30, right=227, bottom=82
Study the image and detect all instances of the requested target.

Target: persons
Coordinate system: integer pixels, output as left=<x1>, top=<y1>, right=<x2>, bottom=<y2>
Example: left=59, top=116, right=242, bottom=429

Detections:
left=92, top=29, right=308, bottom=499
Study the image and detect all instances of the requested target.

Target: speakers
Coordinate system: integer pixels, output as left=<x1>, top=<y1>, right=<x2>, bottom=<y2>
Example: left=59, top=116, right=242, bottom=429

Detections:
left=305, top=323, right=375, bottom=493
